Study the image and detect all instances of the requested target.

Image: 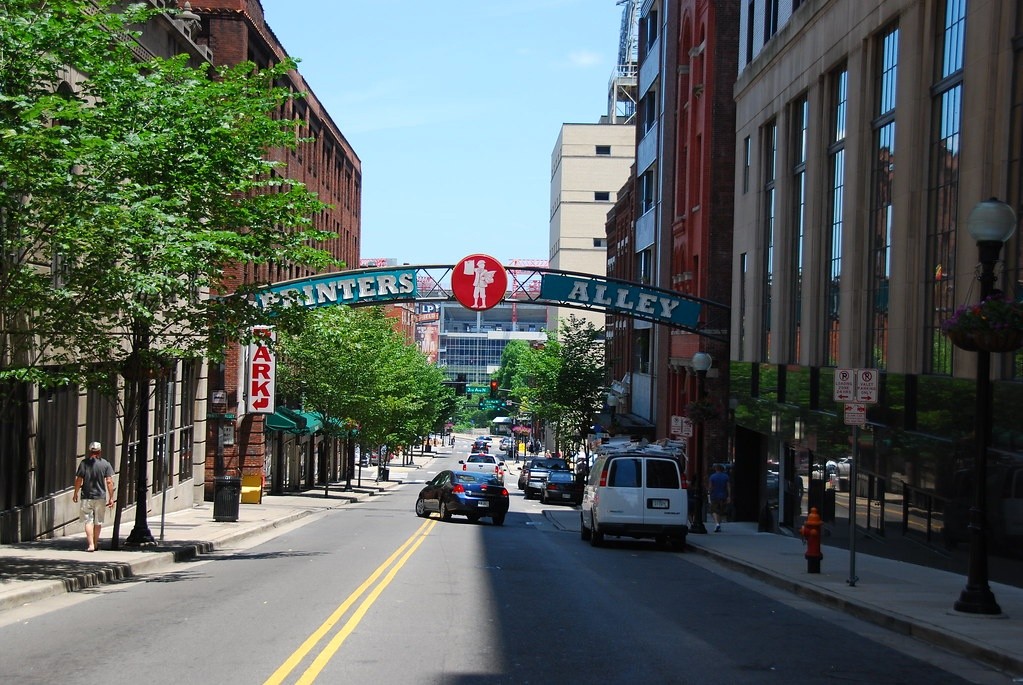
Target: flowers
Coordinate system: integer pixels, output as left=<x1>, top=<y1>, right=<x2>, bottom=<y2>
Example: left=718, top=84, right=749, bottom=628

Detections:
left=941, top=296, right=1023, bottom=335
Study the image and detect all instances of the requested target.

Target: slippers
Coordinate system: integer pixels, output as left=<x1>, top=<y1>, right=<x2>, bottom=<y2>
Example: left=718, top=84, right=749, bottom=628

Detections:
left=85, top=548, right=94, bottom=552
left=94, top=546, right=98, bottom=551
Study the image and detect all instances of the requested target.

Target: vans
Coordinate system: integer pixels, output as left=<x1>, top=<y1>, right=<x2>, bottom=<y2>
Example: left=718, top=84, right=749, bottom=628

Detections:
left=578, top=451, right=688, bottom=553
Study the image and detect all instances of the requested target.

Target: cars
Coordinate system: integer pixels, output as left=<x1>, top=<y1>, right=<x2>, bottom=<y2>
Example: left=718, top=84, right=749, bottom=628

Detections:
left=941, top=506, right=1004, bottom=556
left=415, top=468, right=510, bottom=526
left=371, top=446, right=398, bottom=466
left=767, top=456, right=852, bottom=512
left=498, top=466, right=507, bottom=487
left=550, top=451, right=600, bottom=471
left=540, top=471, right=582, bottom=505
left=517, top=460, right=531, bottom=489
left=472, top=435, right=517, bottom=457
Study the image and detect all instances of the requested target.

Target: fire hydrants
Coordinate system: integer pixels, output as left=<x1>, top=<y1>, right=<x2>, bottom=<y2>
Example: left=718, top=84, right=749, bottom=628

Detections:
left=800, top=508, right=824, bottom=574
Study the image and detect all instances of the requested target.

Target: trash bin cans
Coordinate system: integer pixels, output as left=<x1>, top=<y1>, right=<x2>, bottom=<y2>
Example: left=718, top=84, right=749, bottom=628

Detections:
left=425, top=445, right=431, bottom=452
left=378, top=466, right=389, bottom=481
left=212, top=477, right=242, bottom=522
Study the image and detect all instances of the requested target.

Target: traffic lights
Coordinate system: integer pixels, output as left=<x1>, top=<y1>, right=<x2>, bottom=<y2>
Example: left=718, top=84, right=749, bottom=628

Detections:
left=497, top=406, right=500, bottom=412
left=490, top=380, right=498, bottom=399
left=479, top=397, right=484, bottom=407
left=501, top=397, right=506, bottom=407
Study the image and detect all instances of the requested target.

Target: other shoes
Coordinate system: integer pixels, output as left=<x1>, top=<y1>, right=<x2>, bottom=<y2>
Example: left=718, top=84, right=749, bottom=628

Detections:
left=714, top=524, right=721, bottom=533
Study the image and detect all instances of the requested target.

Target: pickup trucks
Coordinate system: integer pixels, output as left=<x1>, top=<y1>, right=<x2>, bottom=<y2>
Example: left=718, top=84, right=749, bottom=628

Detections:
left=459, top=453, right=504, bottom=481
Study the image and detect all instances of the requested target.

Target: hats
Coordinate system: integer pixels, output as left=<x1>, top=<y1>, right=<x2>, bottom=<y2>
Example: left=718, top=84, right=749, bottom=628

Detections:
left=88, top=442, right=101, bottom=452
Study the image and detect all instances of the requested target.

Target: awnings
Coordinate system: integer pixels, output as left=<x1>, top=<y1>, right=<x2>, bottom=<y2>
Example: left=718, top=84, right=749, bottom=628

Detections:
left=267, top=404, right=350, bottom=439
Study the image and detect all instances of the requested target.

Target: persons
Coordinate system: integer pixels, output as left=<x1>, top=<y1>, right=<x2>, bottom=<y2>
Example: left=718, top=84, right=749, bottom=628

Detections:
left=709, top=466, right=729, bottom=532
left=73, top=441, right=116, bottom=551
left=577, top=460, right=590, bottom=478
left=450, top=436, right=456, bottom=451
left=514, top=438, right=551, bottom=463
left=687, top=474, right=698, bottom=526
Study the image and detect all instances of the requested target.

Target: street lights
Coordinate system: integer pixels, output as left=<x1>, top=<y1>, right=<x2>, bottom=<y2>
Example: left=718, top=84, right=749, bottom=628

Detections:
left=687, top=352, right=712, bottom=534
left=607, top=396, right=619, bottom=438
left=953, top=197, right=1018, bottom=618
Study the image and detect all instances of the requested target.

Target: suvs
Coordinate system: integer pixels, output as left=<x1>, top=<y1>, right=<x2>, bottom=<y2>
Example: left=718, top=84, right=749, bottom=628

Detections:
left=524, top=457, right=570, bottom=499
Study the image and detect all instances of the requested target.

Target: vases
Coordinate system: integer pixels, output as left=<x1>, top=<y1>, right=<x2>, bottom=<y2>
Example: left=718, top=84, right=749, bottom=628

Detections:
left=947, top=329, right=1023, bottom=352
